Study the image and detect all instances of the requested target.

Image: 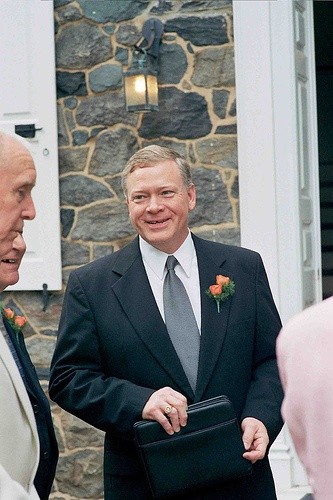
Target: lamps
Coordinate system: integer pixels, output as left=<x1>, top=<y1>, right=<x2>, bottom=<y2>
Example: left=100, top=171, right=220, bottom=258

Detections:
left=121, top=18, right=164, bottom=114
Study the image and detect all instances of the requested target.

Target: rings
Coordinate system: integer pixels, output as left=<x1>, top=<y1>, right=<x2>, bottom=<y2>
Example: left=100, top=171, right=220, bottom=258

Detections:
left=163, top=404, right=175, bottom=415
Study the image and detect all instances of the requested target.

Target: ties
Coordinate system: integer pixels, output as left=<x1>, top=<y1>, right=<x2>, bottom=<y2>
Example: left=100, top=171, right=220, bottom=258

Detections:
left=162, top=255, right=202, bottom=394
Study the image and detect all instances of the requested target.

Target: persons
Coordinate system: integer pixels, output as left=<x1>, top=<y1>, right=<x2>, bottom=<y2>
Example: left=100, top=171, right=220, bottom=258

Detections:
left=276, top=295, right=333, bottom=500
left=0, top=126, right=61, bottom=500
left=48, top=145, right=285, bottom=500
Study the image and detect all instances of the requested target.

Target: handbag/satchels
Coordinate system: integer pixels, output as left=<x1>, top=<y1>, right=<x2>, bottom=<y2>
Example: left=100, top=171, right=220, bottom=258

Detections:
left=132, top=395, right=253, bottom=496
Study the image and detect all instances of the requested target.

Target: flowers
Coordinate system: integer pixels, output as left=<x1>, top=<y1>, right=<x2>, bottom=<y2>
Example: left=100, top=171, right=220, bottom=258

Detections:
left=204, top=274, right=235, bottom=313
left=0, top=303, right=30, bottom=345
